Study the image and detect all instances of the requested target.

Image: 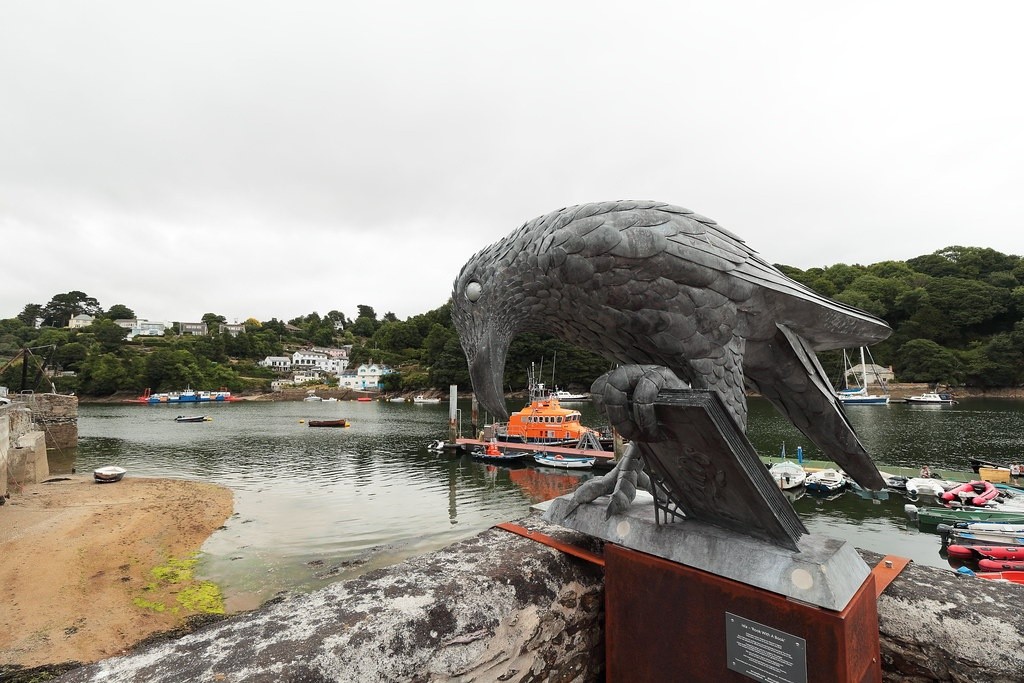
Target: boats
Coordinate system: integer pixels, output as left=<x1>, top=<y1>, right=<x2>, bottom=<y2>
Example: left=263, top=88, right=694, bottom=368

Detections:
left=769, top=460, right=805, bottom=490
left=906, top=478, right=1024, bottom=583
left=140, top=386, right=241, bottom=404
left=301, top=395, right=442, bottom=404
left=471, top=438, right=528, bottom=463
left=804, top=468, right=845, bottom=499
left=176, top=415, right=207, bottom=422
left=308, top=420, right=347, bottom=427
left=967, top=456, right=1024, bottom=474
left=534, top=452, right=596, bottom=468
left=476, top=353, right=628, bottom=446
left=902, top=382, right=960, bottom=405
left=550, top=385, right=589, bottom=399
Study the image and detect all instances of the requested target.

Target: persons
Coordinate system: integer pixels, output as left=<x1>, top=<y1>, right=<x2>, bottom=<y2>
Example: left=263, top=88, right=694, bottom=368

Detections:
left=919, top=465, right=931, bottom=478
left=1010, top=462, right=1020, bottom=486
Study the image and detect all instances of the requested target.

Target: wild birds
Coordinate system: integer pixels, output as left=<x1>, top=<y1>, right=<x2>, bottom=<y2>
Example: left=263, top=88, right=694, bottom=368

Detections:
left=449, top=198, right=889, bottom=522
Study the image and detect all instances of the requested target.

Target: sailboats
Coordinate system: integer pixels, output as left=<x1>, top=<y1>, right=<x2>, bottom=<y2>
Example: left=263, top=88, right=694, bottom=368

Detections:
left=834, top=345, right=891, bottom=406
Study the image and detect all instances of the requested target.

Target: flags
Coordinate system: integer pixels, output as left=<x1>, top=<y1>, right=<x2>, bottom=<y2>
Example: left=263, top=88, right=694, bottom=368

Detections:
left=782, top=444, right=785, bottom=460
